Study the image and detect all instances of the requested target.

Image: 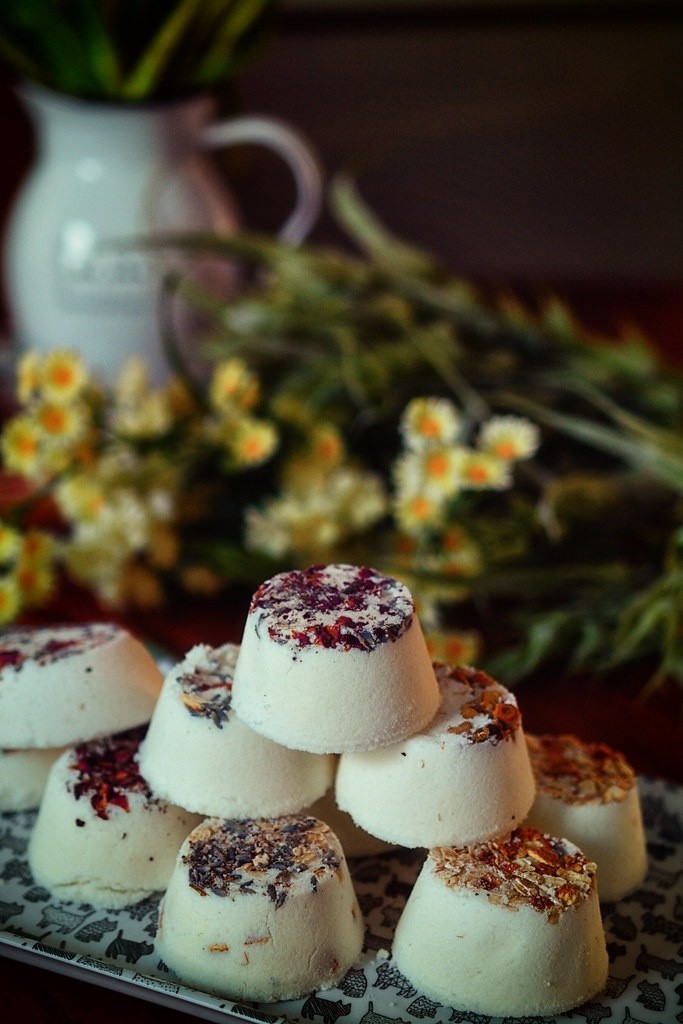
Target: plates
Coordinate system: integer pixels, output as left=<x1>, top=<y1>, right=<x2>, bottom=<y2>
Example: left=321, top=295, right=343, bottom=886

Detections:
left=0, top=774, right=683, bottom=1024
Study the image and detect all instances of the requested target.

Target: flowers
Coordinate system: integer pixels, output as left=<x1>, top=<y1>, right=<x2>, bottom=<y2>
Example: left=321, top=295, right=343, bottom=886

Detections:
left=0, top=179, right=683, bottom=674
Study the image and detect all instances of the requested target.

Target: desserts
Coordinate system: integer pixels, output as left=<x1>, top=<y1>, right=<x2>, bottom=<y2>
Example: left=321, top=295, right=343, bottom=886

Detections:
left=0, top=562, right=652, bottom=1016
